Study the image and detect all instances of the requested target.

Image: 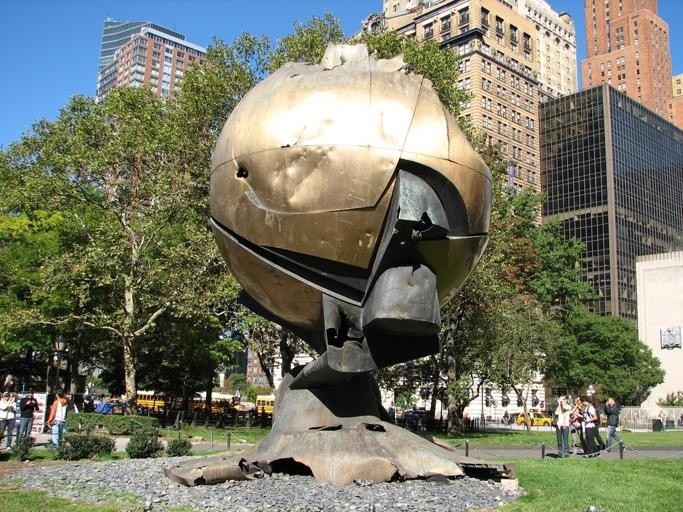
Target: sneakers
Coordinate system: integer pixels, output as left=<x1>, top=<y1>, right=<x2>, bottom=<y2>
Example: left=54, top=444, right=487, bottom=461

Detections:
left=557, top=442, right=627, bottom=461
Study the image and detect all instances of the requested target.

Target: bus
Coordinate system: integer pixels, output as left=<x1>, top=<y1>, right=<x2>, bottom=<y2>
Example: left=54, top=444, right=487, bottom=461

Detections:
left=136, top=391, right=274, bottom=416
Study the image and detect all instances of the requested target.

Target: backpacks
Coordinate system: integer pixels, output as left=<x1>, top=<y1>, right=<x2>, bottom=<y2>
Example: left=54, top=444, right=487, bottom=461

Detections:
left=587, top=404, right=601, bottom=425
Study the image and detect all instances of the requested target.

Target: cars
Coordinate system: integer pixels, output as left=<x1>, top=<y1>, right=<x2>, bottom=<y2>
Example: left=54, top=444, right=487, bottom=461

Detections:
left=508, top=413, right=553, bottom=426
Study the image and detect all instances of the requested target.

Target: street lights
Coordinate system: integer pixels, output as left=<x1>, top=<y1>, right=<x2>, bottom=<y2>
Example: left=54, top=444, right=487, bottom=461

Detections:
left=55, top=333, right=65, bottom=380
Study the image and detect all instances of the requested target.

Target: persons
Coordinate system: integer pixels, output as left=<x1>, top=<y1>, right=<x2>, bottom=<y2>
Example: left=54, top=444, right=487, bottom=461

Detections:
left=502, top=411, right=510, bottom=426
left=529, top=410, right=535, bottom=426
left=406, top=407, right=470, bottom=433
left=604, top=397, right=625, bottom=453
left=0, top=384, right=131, bottom=450
left=554, top=394, right=605, bottom=458
